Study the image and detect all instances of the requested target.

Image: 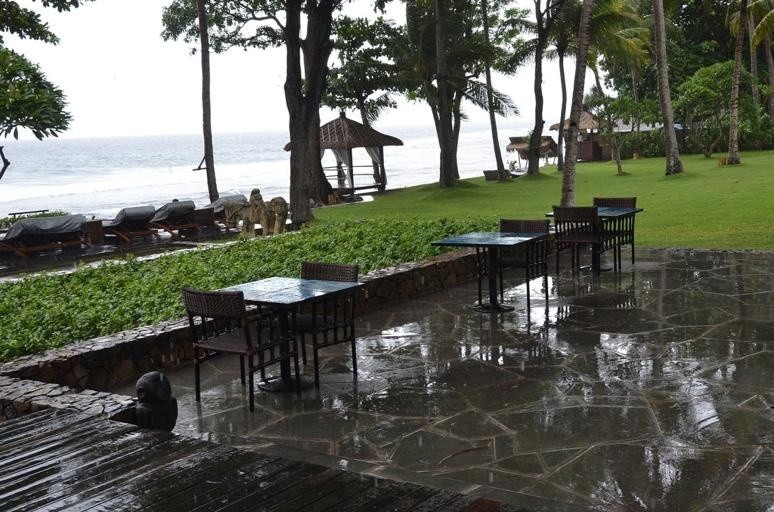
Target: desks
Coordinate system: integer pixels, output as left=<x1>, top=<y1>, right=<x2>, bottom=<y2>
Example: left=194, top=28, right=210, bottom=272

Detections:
left=433, top=228, right=552, bottom=312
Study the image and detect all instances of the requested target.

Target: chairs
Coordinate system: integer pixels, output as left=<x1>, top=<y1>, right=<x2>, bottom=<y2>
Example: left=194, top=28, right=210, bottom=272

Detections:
left=179, top=260, right=361, bottom=416
left=499, top=193, right=640, bottom=317
left=0, top=192, right=250, bottom=273
